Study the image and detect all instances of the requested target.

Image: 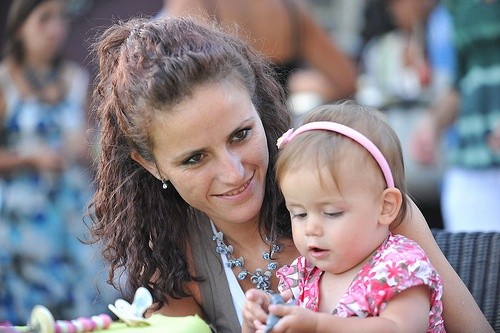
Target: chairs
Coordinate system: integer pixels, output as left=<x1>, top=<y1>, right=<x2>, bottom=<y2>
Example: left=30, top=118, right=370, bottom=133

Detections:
left=434, top=230, right=500, bottom=333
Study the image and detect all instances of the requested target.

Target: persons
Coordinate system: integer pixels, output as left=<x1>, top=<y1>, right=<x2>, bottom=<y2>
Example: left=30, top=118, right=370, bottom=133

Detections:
left=1, top=0, right=133, bottom=326
left=157, top=0, right=500, bottom=235
left=76, top=15, right=494, bottom=333
left=242, top=103, right=446, bottom=332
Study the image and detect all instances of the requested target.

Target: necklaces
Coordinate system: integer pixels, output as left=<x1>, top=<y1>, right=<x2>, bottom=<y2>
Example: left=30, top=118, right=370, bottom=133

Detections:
left=209, top=218, right=280, bottom=297
